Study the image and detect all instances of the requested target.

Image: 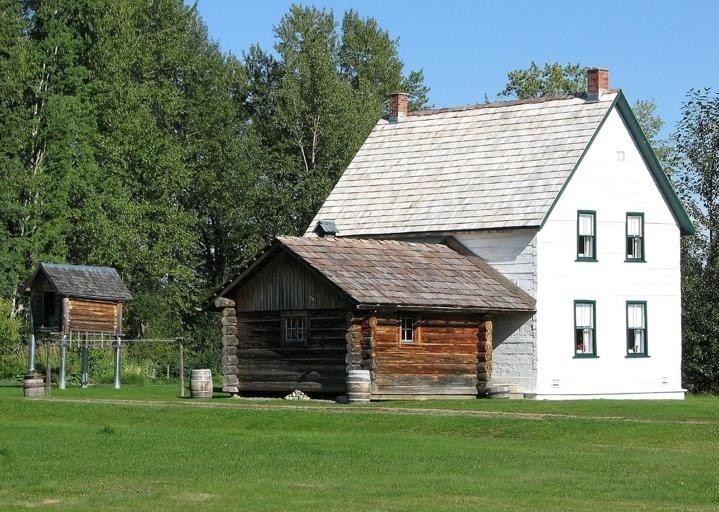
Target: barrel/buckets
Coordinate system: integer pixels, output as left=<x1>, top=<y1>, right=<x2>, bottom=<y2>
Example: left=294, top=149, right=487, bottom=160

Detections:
left=189, top=369, right=214, bottom=398
left=345, top=369, right=370, bottom=403
left=23, top=376, right=45, bottom=398
